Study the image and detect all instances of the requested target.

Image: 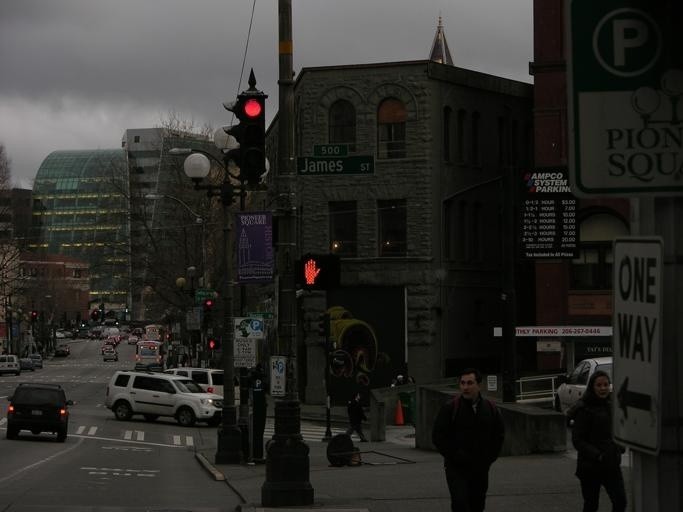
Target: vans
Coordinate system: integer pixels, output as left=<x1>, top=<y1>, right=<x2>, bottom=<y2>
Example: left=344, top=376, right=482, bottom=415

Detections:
left=161, top=366, right=240, bottom=406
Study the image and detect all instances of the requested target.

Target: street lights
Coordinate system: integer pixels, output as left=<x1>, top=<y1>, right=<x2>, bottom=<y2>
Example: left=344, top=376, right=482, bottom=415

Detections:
left=168, top=148, right=256, bottom=463
left=143, top=192, right=209, bottom=292
left=175, top=264, right=210, bottom=358
left=182, top=125, right=270, bottom=465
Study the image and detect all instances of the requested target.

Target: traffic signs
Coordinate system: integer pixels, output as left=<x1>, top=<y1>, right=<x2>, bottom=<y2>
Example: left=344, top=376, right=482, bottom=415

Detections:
left=297, top=155, right=374, bottom=176
left=610, top=235, right=665, bottom=457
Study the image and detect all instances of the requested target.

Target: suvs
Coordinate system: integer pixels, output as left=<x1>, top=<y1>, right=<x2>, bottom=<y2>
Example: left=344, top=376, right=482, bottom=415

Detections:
left=103, top=370, right=223, bottom=427
left=6, top=382, right=72, bottom=442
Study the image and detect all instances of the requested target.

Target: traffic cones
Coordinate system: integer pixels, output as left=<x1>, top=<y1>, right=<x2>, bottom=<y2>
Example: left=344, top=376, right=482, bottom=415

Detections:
left=392, top=399, right=407, bottom=426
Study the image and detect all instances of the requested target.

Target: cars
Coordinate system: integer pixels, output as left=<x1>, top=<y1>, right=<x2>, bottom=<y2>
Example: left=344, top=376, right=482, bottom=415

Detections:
left=554, top=357, right=613, bottom=421
left=0, top=318, right=166, bottom=376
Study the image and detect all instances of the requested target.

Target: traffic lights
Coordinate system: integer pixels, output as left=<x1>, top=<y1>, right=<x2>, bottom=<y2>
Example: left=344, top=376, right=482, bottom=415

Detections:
left=31, top=310, right=37, bottom=322
left=317, top=312, right=329, bottom=335
left=206, top=338, right=220, bottom=350
left=222, top=96, right=244, bottom=173
left=299, top=253, right=341, bottom=292
left=92, top=311, right=97, bottom=321
left=205, top=300, right=213, bottom=312
left=240, top=93, right=265, bottom=178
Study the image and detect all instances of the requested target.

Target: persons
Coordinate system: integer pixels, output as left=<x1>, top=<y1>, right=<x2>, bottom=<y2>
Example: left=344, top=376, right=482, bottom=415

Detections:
left=390, top=379, right=398, bottom=388
left=405, top=374, right=415, bottom=385
left=431, top=367, right=507, bottom=510
left=564, top=368, right=628, bottom=511
left=395, top=375, right=405, bottom=385
left=344, top=388, right=369, bottom=441
